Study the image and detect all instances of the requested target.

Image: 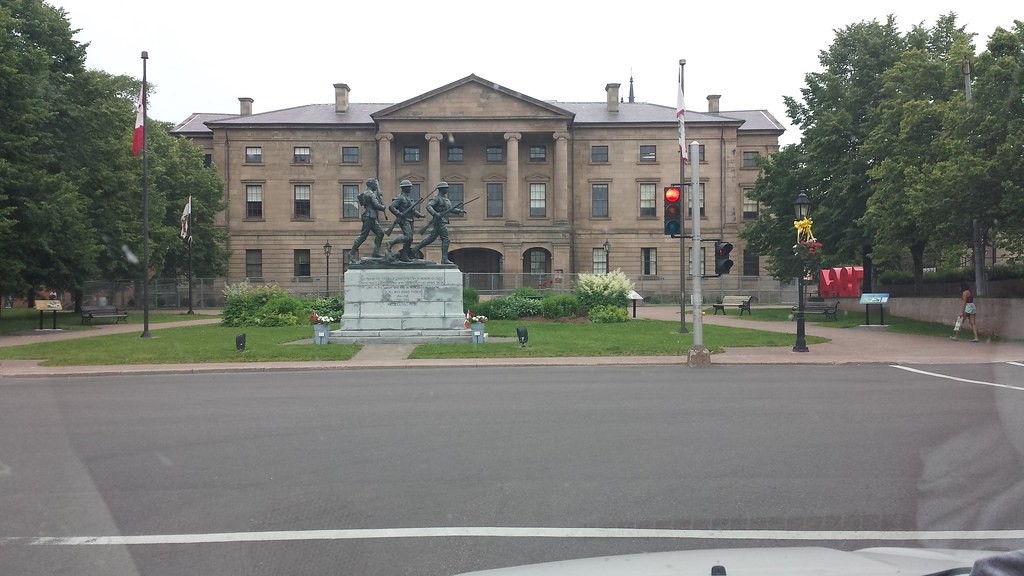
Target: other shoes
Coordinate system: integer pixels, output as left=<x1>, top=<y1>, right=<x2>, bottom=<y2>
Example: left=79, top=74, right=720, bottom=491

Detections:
left=950, top=335, right=958, bottom=341
left=970, top=338, right=979, bottom=342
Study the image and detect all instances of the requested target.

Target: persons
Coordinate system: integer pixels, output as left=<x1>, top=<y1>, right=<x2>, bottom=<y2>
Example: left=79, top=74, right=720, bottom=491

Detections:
left=414, top=181, right=467, bottom=263
left=386, top=180, right=426, bottom=262
left=950, top=284, right=979, bottom=343
left=49, top=288, right=57, bottom=300
left=349, top=178, right=386, bottom=263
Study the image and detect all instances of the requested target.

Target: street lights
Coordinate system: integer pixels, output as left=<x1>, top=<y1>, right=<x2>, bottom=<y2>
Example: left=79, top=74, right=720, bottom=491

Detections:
left=603, top=238, right=611, bottom=275
left=793, top=189, right=812, bottom=352
left=323, top=240, right=332, bottom=298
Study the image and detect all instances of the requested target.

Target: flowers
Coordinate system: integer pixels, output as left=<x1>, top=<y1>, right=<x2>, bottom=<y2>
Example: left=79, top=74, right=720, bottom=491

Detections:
left=464, top=309, right=488, bottom=328
left=309, top=311, right=336, bottom=325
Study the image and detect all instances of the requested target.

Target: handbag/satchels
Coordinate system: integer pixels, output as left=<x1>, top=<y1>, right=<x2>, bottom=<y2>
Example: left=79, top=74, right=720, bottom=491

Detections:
left=953, top=315, right=964, bottom=332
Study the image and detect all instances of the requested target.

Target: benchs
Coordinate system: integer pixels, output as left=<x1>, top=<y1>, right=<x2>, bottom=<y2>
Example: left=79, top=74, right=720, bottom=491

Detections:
left=791, top=300, right=840, bottom=322
left=712, top=295, right=753, bottom=316
left=79, top=304, right=128, bottom=324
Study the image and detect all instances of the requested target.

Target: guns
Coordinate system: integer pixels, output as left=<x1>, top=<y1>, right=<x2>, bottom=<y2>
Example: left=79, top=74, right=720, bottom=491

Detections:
left=384, top=187, right=438, bottom=237
left=419, top=196, right=480, bottom=236
left=375, top=176, right=389, bottom=220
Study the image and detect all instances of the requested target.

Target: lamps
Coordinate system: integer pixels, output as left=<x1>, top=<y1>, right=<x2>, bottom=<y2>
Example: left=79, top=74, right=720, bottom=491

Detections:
left=236, top=332, right=246, bottom=351
left=319, top=333, right=325, bottom=337
left=517, top=326, right=528, bottom=347
left=474, top=331, right=481, bottom=335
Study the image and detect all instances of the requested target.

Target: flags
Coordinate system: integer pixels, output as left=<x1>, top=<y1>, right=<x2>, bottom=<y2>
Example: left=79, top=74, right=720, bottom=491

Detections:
left=181, top=196, right=191, bottom=238
left=132, top=84, right=143, bottom=157
left=677, top=66, right=688, bottom=165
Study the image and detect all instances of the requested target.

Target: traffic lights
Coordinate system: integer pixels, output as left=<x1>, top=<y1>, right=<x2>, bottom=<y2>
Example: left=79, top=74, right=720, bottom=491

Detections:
left=664, top=187, right=681, bottom=235
left=714, top=242, right=734, bottom=274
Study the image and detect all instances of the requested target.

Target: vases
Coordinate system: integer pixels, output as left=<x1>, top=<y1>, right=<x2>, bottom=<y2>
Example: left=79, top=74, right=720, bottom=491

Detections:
left=469, top=323, right=484, bottom=344
left=313, top=324, right=328, bottom=344
